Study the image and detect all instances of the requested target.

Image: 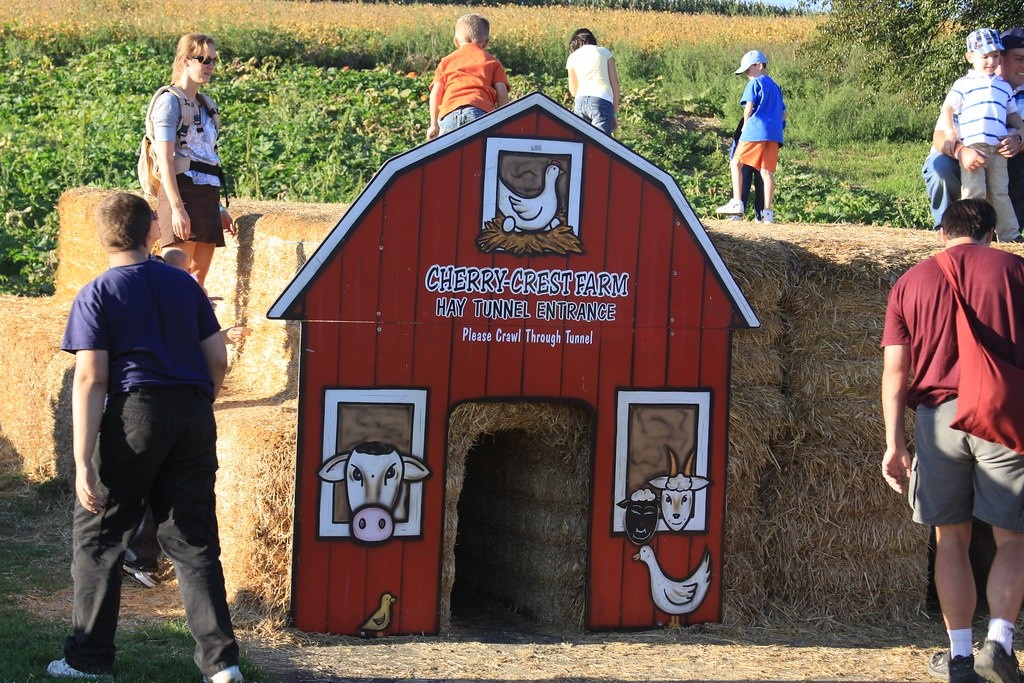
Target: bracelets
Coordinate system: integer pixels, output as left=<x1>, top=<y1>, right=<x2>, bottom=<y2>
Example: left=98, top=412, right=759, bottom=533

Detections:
left=954, top=144, right=966, bottom=160
left=219, top=206, right=225, bottom=213
left=613, top=112, right=618, bottom=118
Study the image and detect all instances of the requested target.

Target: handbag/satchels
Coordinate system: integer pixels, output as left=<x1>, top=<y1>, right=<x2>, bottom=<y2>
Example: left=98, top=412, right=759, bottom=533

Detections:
left=935, top=247, right=1024, bottom=457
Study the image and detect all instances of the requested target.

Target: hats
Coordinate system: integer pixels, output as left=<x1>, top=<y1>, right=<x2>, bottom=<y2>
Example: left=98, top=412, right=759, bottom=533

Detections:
left=1000, top=26, right=1024, bottom=48
left=734, top=50, right=768, bottom=75
left=966, top=28, right=1004, bottom=56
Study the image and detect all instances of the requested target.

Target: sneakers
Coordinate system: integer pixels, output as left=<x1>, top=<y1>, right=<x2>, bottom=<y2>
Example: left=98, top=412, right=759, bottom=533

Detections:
left=202, top=665, right=244, bottom=683
left=46, top=657, right=113, bottom=680
left=730, top=215, right=743, bottom=220
left=756, top=209, right=773, bottom=223
left=974, top=639, right=1024, bottom=683
left=122, top=564, right=162, bottom=588
left=927, top=646, right=974, bottom=683
left=715, top=198, right=744, bottom=215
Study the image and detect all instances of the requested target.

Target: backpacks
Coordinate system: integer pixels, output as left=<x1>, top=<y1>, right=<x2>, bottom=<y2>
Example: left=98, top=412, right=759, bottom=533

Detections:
left=137, top=85, right=219, bottom=198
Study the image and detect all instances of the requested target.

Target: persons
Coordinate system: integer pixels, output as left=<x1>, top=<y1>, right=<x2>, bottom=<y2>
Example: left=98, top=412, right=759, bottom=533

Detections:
left=46, top=191, right=247, bottom=683
left=565, top=28, right=620, bottom=138
left=119, top=32, right=236, bottom=589
left=923, top=27, right=1024, bottom=243
left=715, top=50, right=787, bottom=222
left=880, top=197, right=1024, bottom=683
left=426, top=13, right=511, bottom=142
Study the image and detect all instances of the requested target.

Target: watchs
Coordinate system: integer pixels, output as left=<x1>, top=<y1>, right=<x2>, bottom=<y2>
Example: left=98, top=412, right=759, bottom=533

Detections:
left=1015, top=134, right=1022, bottom=145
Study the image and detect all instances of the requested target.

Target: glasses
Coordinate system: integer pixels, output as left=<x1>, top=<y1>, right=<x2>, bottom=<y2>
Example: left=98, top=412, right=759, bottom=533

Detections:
left=187, top=55, right=217, bottom=66
